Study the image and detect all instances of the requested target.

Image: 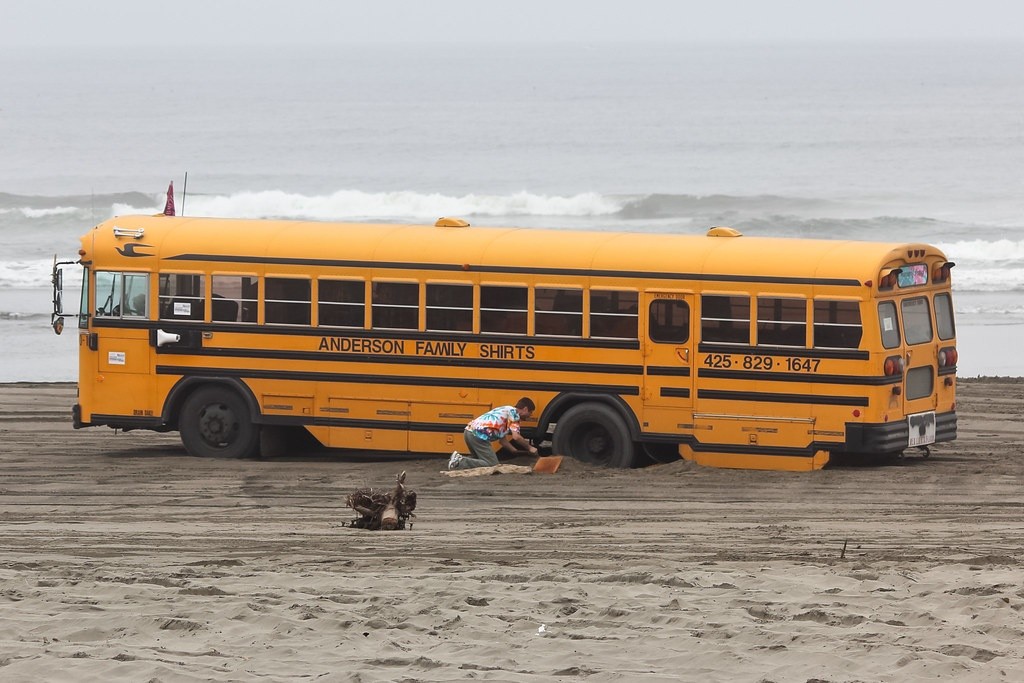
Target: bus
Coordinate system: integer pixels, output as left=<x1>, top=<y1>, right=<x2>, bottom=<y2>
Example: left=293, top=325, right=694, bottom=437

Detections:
left=51, top=214, right=961, bottom=472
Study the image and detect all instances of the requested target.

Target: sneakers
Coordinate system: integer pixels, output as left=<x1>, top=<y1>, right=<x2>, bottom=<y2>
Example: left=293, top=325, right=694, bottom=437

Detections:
left=448, top=450, right=462, bottom=470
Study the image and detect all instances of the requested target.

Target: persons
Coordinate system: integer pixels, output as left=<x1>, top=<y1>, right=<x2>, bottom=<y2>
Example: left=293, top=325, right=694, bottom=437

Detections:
left=446, top=397, right=538, bottom=470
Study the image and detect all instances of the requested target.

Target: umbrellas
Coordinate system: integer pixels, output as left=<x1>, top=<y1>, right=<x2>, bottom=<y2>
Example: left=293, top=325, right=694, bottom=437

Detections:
left=159, top=184, right=176, bottom=302
